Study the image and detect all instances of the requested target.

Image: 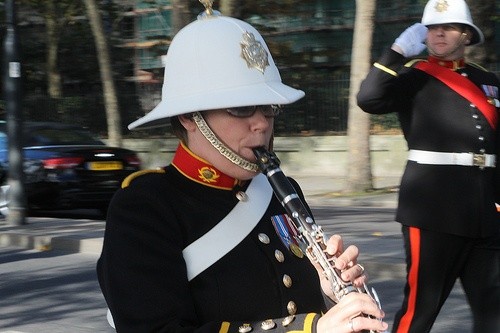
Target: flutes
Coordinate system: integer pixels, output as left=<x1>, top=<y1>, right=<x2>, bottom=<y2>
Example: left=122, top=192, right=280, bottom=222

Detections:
left=248, top=144, right=390, bottom=333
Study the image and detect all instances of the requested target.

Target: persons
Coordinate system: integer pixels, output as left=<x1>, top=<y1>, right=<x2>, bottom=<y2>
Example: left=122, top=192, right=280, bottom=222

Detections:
left=95, top=0, right=389, bottom=333
left=356, top=0, right=500, bottom=333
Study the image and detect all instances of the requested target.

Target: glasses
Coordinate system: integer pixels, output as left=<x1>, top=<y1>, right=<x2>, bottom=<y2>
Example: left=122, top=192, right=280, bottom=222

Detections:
left=227, top=104, right=286, bottom=118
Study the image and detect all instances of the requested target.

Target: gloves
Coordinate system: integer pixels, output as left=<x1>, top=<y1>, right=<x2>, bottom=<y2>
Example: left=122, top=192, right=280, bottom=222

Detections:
left=394, top=23, right=429, bottom=58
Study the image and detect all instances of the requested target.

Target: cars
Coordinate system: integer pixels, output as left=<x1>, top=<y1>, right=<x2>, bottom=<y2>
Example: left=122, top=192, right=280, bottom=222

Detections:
left=0, top=120, right=144, bottom=214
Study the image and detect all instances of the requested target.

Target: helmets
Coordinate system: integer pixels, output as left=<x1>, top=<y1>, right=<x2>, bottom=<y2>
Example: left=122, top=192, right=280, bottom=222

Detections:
left=421, top=0, right=484, bottom=48
left=127, top=0, right=305, bottom=131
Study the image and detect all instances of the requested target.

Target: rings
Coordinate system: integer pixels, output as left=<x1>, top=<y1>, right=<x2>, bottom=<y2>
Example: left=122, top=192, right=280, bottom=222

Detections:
left=347, top=320, right=354, bottom=331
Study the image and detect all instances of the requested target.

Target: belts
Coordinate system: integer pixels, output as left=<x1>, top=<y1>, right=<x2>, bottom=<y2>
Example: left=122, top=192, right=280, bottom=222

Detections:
left=406, top=149, right=496, bottom=168
